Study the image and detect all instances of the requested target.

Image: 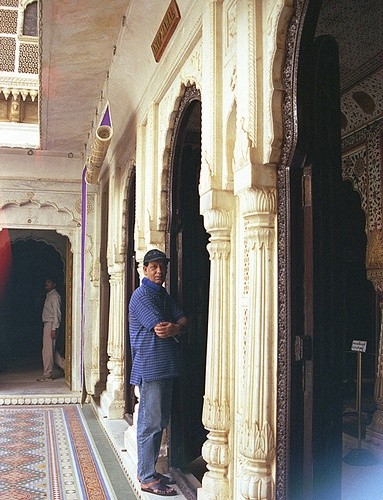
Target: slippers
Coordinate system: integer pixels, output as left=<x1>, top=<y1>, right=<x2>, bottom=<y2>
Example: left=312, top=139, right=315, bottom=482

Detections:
left=157, top=473, right=176, bottom=485
left=141, top=482, right=175, bottom=496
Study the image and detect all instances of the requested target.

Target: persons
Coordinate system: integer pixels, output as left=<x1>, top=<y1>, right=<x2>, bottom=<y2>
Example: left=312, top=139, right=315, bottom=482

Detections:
left=127, top=248, right=190, bottom=498
left=36, top=276, right=66, bottom=382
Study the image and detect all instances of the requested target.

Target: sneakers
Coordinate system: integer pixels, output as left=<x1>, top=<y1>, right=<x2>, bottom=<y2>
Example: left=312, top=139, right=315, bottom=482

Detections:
left=36, top=373, right=54, bottom=382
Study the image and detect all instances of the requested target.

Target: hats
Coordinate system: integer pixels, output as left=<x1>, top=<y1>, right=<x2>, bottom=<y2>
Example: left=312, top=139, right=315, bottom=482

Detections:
left=143, top=249, right=172, bottom=266
